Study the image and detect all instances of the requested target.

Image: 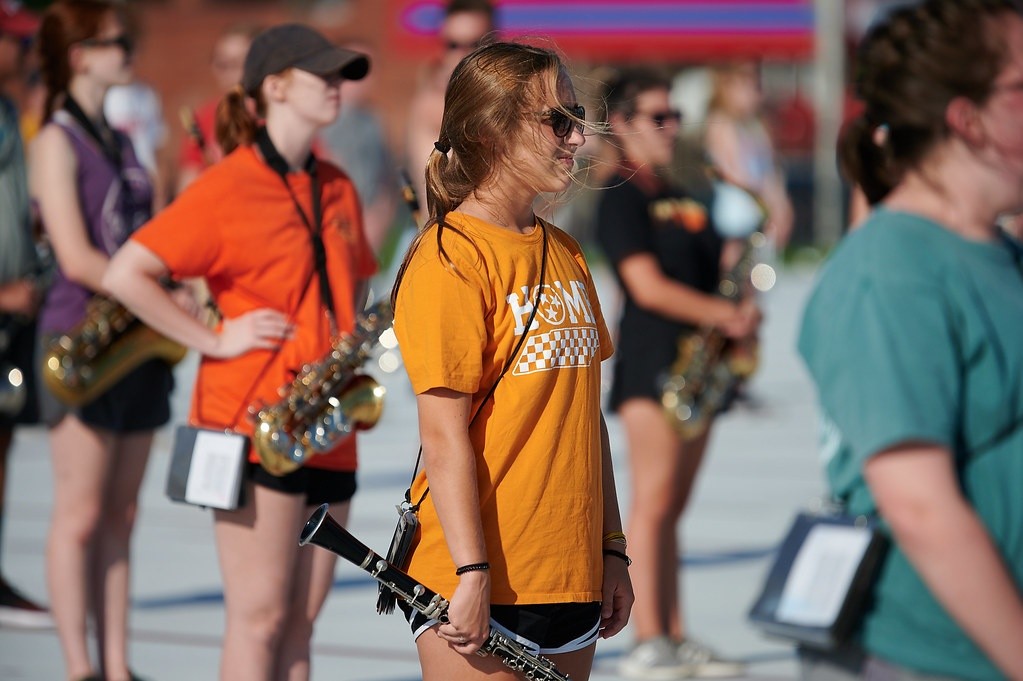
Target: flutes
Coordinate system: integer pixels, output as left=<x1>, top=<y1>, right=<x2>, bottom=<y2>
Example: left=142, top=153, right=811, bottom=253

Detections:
left=298, top=503, right=570, bottom=681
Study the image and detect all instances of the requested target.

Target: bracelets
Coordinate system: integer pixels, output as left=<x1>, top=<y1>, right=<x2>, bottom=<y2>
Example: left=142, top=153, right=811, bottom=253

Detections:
left=605, top=547, right=635, bottom=568
left=455, top=560, right=489, bottom=574
left=603, top=531, right=630, bottom=547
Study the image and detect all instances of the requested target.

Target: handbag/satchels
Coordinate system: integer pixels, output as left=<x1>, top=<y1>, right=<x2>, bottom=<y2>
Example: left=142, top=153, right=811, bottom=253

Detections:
left=372, top=508, right=417, bottom=617
left=166, top=425, right=249, bottom=511
left=748, top=516, right=895, bottom=661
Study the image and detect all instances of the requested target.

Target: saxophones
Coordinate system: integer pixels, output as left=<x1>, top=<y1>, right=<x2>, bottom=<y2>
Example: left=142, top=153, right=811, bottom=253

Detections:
left=245, top=167, right=424, bottom=477
left=44, top=105, right=215, bottom=408
left=663, top=140, right=793, bottom=441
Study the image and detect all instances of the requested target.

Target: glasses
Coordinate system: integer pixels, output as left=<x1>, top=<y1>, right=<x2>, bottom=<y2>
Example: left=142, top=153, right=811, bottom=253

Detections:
left=635, top=109, right=682, bottom=126
left=3, top=36, right=33, bottom=54
left=522, top=105, right=585, bottom=138
left=83, top=33, right=134, bottom=51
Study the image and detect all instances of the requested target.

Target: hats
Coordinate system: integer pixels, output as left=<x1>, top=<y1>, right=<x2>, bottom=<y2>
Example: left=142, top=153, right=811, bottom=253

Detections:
left=242, top=23, right=369, bottom=81
left=0, top=0, right=43, bottom=34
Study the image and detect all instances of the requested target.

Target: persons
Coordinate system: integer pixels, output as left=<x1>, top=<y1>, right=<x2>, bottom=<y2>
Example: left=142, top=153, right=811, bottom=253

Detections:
left=1, top=0, right=1023, bottom=681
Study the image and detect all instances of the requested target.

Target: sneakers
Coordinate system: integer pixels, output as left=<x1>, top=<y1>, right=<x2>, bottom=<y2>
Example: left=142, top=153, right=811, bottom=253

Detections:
left=616, top=635, right=745, bottom=681
